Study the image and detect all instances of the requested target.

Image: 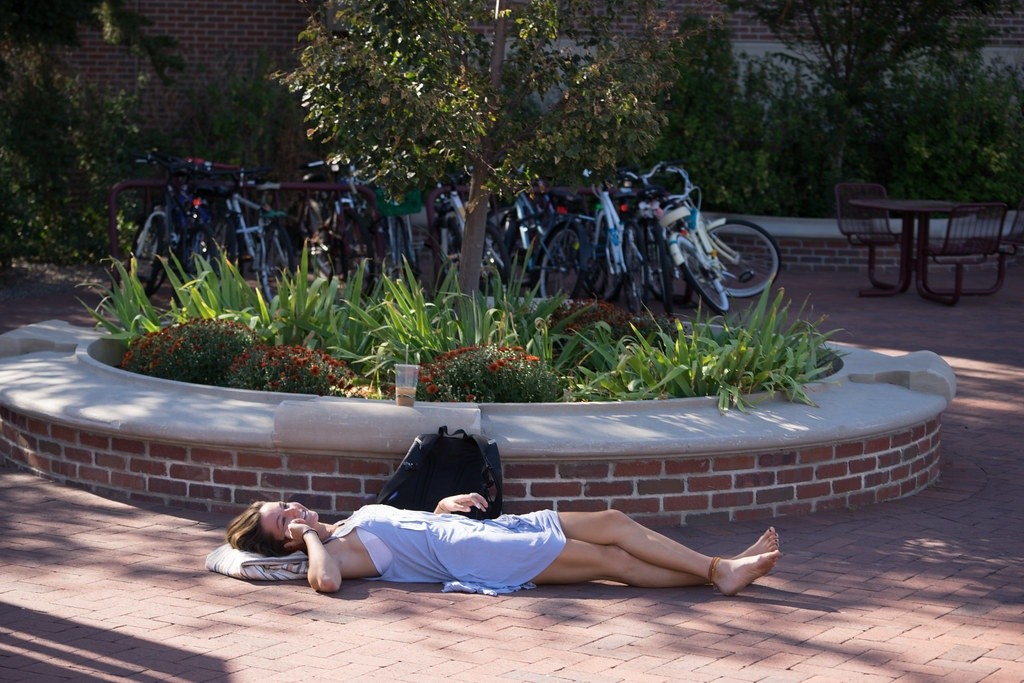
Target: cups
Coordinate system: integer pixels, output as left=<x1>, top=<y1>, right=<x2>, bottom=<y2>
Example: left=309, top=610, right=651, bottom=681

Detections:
left=393, top=362, right=420, bottom=405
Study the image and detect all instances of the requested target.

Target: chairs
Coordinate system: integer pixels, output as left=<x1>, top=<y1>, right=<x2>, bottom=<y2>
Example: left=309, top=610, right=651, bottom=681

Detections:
left=835, top=181, right=920, bottom=296
left=918, top=199, right=1008, bottom=306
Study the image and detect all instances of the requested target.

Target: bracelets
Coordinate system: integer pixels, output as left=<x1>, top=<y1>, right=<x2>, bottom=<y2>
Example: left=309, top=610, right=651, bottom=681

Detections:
left=438, top=500, right=447, bottom=514
left=301, top=528, right=319, bottom=544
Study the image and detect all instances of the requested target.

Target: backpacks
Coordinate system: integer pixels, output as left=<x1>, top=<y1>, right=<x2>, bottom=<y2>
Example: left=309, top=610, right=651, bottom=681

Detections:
left=379, top=422, right=502, bottom=519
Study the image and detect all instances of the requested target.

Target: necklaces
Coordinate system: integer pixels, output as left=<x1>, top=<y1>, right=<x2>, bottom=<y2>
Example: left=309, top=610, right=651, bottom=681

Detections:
left=323, top=522, right=345, bottom=540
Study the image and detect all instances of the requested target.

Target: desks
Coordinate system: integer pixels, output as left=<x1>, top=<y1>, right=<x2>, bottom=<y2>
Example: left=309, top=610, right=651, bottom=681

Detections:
left=845, top=197, right=985, bottom=307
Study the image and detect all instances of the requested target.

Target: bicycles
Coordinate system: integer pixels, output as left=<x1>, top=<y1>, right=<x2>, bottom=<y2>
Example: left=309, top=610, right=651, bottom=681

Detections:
left=126, top=152, right=782, bottom=325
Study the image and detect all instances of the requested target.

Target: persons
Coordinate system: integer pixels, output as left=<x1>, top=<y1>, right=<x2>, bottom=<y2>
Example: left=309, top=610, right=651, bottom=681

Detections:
left=225, top=491, right=783, bottom=597
